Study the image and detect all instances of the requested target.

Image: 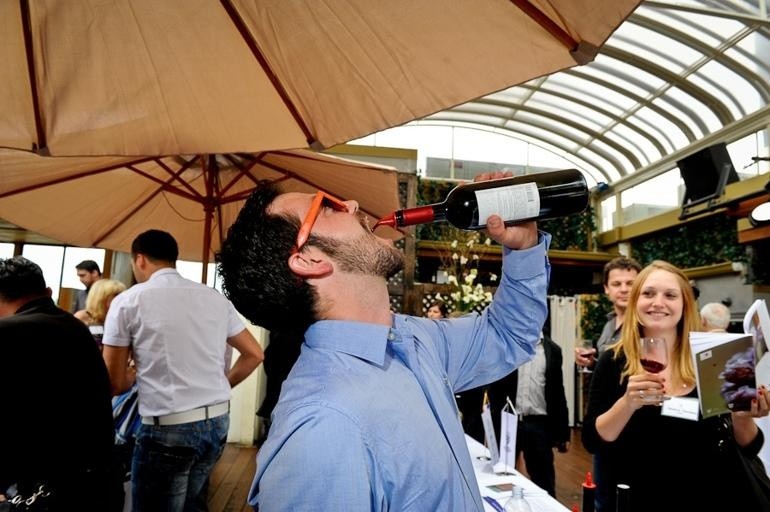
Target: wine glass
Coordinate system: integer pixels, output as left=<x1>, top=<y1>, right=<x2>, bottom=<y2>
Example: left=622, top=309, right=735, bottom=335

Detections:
left=575, top=336, right=594, bottom=374
left=636, top=335, right=673, bottom=402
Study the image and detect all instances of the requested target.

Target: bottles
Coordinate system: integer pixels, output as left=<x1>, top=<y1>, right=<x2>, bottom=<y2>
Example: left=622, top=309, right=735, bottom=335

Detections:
left=504, top=486, right=532, bottom=512
left=392, top=167, right=591, bottom=235
left=581, top=470, right=598, bottom=511
left=616, top=483, right=633, bottom=512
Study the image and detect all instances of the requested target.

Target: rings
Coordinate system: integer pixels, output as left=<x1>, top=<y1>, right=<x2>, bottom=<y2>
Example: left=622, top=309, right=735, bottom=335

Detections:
left=640, top=390, right=643, bottom=397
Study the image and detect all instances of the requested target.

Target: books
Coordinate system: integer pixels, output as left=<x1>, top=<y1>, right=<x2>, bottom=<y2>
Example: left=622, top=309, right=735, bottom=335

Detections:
left=689, top=300, right=770, bottom=418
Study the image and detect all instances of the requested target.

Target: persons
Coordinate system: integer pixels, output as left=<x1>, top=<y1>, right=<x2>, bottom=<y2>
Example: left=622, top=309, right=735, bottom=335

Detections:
left=102, top=228, right=265, bottom=512
left=428, top=301, right=446, bottom=321
left=488, top=328, right=571, bottom=497
left=581, top=260, right=770, bottom=512
left=698, top=301, right=730, bottom=334
left=217, top=172, right=552, bottom=512
left=0, top=256, right=140, bottom=512
left=575, top=255, right=644, bottom=371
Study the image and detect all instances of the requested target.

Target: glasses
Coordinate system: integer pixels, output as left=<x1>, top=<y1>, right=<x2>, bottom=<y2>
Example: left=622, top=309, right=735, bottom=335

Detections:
left=290, top=189, right=347, bottom=256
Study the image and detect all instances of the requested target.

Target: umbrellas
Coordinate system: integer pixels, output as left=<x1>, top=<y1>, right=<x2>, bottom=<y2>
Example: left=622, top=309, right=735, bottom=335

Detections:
left=0, top=147, right=399, bottom=284
left=0, top=0, right=644, bottom=156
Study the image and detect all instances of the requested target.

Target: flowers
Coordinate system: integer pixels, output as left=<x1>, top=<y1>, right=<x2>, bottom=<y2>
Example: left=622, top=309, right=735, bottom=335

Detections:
left=427, top=221, right=498, bottom=319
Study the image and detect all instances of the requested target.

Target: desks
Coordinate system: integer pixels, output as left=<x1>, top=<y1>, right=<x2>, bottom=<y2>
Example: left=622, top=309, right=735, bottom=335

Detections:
left=463, top=431, right=572, bottom=511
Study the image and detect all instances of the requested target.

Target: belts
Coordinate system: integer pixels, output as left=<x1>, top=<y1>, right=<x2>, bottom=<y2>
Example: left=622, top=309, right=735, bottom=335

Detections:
left=140, top=400, right=231, bottom=427
